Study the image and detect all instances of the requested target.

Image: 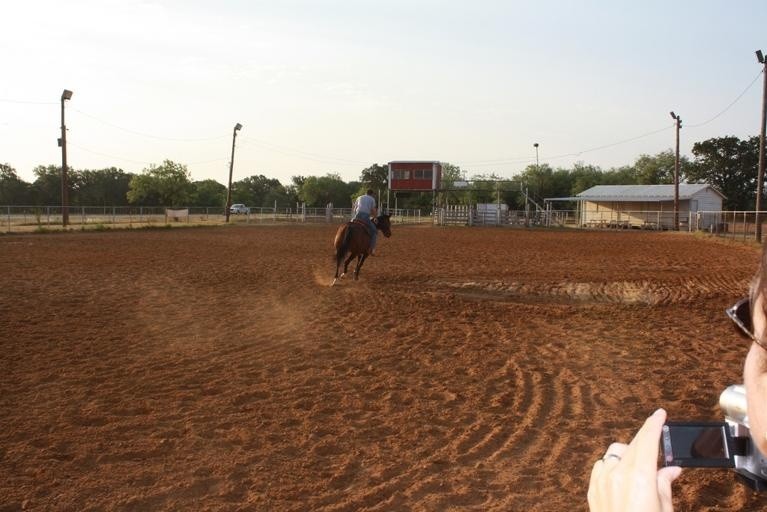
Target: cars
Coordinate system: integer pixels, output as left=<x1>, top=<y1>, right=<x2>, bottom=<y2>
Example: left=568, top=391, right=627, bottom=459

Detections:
left=228, top=202, right=250, bottom=216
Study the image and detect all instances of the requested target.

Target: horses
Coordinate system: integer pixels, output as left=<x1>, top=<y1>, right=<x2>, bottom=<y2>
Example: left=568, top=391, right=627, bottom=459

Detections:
left=332, top=211, right=393, bottom=280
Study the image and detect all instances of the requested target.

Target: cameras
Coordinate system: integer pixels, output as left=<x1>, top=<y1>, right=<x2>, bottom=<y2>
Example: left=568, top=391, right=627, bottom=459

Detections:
left=660, top=383, right=766, bottom=489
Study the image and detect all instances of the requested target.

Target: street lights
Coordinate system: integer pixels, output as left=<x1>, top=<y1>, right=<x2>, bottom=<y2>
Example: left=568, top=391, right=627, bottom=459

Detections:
left=531, top=143, right=539, bottom=202
left=752, top=51, right=766, bottom=243
left=225, top=122, right=243, bottom=223
left=669, top=111, right=681, bottom=232
left=57, top=87, right=75, bottom=231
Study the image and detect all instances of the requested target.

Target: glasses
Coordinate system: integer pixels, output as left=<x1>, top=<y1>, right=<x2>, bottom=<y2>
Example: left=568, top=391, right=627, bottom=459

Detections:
left=725, top=297, right=767, bottom=352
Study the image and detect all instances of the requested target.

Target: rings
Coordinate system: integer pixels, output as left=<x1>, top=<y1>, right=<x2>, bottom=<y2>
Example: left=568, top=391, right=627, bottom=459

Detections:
left=603, top=453, right=621, bottom=461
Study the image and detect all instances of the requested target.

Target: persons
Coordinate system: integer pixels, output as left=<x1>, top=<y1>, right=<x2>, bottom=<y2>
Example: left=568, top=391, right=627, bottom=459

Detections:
left=351, top=189, right=378, bottom=257
left=586, top=240, right=767, bottom=512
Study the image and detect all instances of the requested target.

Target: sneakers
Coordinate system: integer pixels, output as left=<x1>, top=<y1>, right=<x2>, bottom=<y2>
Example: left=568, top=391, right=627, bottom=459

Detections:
left=370, top=248, right=376, bottom=255
